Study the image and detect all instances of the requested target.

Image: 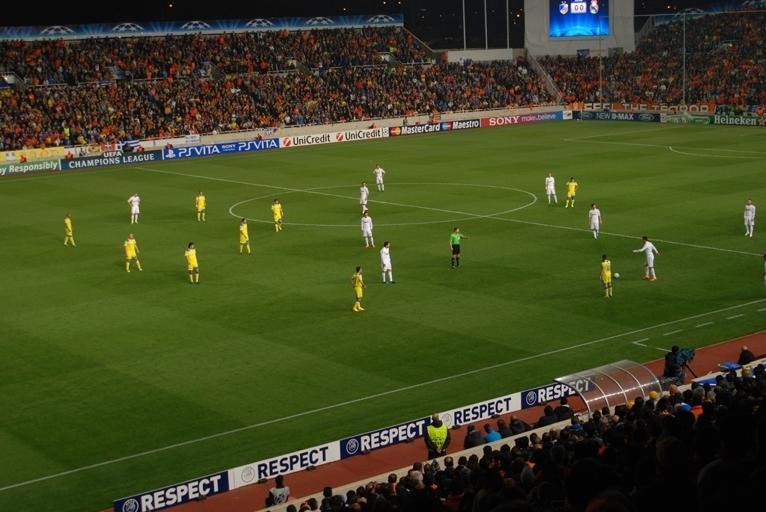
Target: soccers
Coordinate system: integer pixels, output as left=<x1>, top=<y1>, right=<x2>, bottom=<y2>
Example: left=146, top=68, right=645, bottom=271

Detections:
left=613, top=273, right=619, bottom=279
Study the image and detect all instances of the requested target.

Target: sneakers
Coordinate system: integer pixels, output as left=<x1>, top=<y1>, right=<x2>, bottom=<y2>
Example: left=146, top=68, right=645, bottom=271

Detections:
left=365, top=243, right=369, bottom=248
left=138, top=268, right=142, bottom=271
left=126, top=270, right=130, bottom=272
left=650, top=276, right=657, bottom=281
left=352, top=307, right=364, bottom=312
left=642, top=275, right=649, bottom=280
left=371, top=244, right=375, bottom=248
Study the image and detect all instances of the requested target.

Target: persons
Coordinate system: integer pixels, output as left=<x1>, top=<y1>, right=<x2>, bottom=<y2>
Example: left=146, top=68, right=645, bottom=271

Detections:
left=632, top=236, right=659, bottom=283
left=286, top=363, right=766, bottom=512
left=380, top=240, right=396, bottom=284
left=587, top=202, right=604, bottom=240
left=359, top=181, right=370, bottom=214
left=264, top=475, right=292, bottom=507
left=563, top=177, right=580, bottom=210
left=544, top=170, right=560, bottom=209
left=350, top=265, right=367, bottom=313
left=372, top=163, right=386, bottom=192
left=122, top=232, right=144, bottom=273
left=127, top=192, right=141, bottom=226
left=448, top=227, right=469, bottom=271
left=238, top=218, right=253, bottom=254
left=184, top=242, right=200, bottom=286
left=360, top=212, right=376, bottom=248
left=662, top=343, right=682, bottom=377
left=61, top=211, right=78, bottom=247
left=270, top=199, right=284, bottom=234
left=599, top=254, right=613, bottom=301
left=0, top=12, right=764, bottom=150
left=737, top=341, right=755, bottom=364
left=741, top=198, right=757, bottom=240
left=194, top=191, right=208, bottom=223
